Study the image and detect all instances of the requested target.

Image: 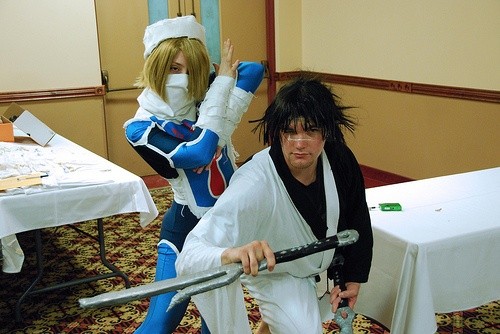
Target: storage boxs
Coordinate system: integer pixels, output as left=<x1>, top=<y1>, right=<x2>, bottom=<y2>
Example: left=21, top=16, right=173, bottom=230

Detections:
left=0, top=102, right=56, bottom=147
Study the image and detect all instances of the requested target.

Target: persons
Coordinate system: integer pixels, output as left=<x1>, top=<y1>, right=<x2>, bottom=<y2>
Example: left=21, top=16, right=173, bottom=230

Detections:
left=122, top=15, right=267, bottom=334
left=175, top=73, right=374, bottom=334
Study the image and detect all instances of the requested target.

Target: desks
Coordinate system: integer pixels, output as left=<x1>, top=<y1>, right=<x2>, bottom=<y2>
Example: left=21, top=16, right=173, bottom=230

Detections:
left=355, top=167, right=500, bottom=334
left=0, top=126, right=159, bottom=322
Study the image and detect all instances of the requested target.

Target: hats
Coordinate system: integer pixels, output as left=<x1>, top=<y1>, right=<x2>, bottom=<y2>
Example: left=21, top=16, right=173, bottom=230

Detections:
left=142, top=15, right=205, bottom=60
